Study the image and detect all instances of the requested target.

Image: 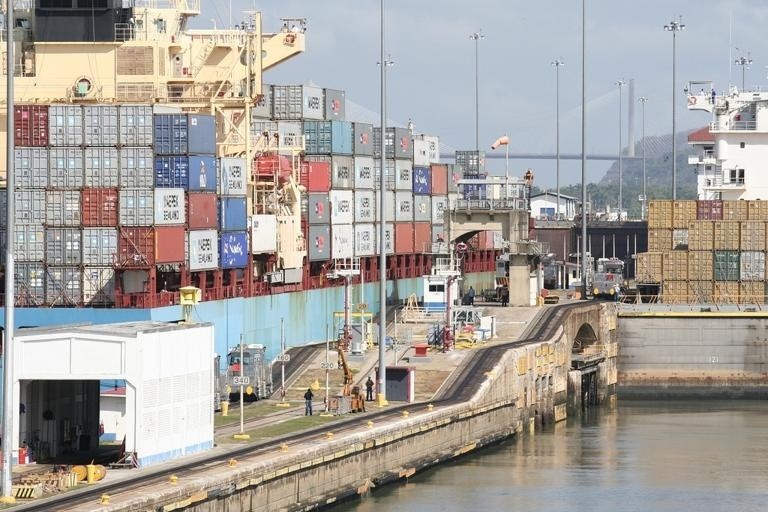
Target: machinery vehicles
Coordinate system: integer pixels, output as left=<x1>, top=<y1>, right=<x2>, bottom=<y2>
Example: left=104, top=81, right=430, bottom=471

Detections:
left=213, top=343, right=272, bottom=411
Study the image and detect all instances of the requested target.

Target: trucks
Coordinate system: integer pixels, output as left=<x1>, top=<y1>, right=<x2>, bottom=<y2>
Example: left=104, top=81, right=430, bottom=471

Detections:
left=591, top=257, right=625, bottom=296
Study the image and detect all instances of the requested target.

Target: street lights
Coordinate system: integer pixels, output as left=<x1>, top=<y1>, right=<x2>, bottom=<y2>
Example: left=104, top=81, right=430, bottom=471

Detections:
left=614, top=76, right=648, bottom=221
left=375, top=53, right=395, bottom=407
left=551, top=59, right=564, bottom=221
left=664, top=21, right=686, bottom=249
left=735, top=57, right=752, bottom=92
left=468, top=29, right=487, bottom=180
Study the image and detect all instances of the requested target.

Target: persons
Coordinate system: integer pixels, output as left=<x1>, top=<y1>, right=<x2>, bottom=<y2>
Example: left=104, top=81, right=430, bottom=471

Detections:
left=281, top=22, right=298, bottom=34
left=500, top=285, right=507, bottom=307
left=364, top=377, right=374, bottom=401
left=468, top=285, right=475, bottom=304
left=303, top=389, right=313, bottom=416
left=699, top=88, right=717, bottom=104
left=480, top=288, right=485, bottom=301
left=614, top=283, right=626, bottom=303
